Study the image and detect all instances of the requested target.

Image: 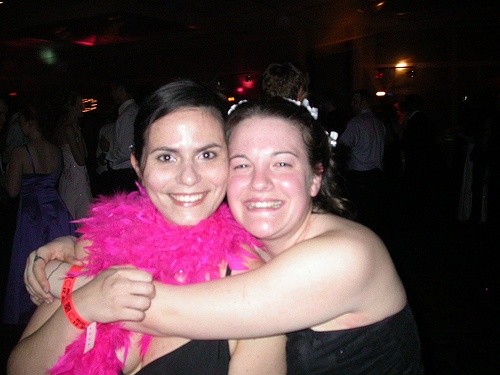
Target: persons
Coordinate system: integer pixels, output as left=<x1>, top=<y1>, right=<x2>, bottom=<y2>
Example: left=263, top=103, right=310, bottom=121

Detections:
left=23, top=95, right=421, bottom=375
left=6, top=79, right=288, bottom=375
left=0, top=62, right=500, bottom=350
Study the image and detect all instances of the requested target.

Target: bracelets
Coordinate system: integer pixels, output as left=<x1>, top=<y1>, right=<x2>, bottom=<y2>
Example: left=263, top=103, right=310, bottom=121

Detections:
left=47, top=262, right=65, bottom=280
left=61, top=294, right=92, bottom=331
left=61, top=265, right=85, bottom=300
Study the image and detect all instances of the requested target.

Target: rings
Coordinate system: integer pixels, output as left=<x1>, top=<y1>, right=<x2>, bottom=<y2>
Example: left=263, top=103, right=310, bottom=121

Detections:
left=33, top=255, right=47, bottom=268
